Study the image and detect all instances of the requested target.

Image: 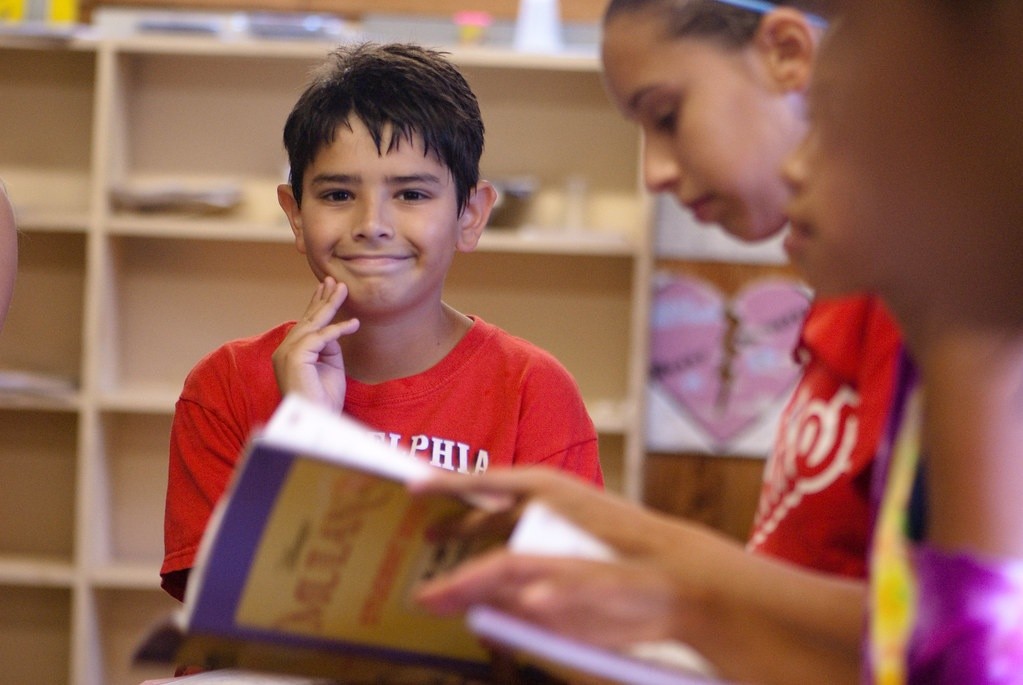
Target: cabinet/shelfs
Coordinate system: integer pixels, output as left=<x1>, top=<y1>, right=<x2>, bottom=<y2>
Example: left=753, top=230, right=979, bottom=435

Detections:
left=0, top=27, right=655, bottom=685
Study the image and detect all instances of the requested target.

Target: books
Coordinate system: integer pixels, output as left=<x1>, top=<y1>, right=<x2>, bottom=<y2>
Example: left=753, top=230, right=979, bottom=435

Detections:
left=115, top=176, right=239, bottom=213
left=467, top=495, right=727, bottom=685
left=131, top=391, right=538, bottom=684
left=0, top=0, right=599, bottom=53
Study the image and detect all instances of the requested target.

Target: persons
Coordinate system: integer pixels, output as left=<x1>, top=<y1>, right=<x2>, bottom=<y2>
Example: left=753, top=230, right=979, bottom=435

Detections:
left=421, top=0, right=1023, bottom=685
left=160, top=38, right=605, bottom=685
